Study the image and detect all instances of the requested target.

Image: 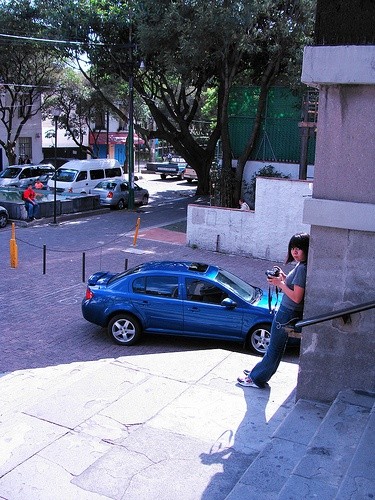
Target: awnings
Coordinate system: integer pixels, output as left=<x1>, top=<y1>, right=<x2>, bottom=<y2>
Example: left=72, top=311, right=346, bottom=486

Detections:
left=89, top=132, right=145, bottom=145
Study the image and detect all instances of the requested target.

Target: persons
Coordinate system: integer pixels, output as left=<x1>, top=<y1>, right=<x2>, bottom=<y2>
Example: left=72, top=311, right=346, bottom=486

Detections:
left=239, top=198, right=250, bottom=210
left=18, top=155, right=24, bottom=165
left=35, top=179, right=44, bottom=190
left=24, top=183, right=44, bottom=221
left=24, top=155, right=30, bottom=164
left=237, top=232, right=310, bottom=388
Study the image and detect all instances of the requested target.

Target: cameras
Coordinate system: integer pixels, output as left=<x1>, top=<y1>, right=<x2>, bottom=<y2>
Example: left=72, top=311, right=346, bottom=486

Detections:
left=265, top=267, right=280, bottom=279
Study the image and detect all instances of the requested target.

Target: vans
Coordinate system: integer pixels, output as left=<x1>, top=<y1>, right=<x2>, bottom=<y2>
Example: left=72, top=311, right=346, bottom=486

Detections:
left=45, top=158, right=125, bottom=195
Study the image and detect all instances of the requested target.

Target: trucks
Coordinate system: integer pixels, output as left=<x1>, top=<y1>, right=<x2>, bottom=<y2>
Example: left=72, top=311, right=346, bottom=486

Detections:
left=155, top=157, right=187, bottom=181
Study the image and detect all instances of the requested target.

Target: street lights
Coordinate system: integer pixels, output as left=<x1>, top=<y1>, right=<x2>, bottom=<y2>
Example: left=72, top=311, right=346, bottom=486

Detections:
left=50, top=105, right=62, bottom=224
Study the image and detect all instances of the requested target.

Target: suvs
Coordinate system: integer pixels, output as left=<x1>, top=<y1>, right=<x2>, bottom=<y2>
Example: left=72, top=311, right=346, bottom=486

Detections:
left=0, top=163, right=56, bottom=190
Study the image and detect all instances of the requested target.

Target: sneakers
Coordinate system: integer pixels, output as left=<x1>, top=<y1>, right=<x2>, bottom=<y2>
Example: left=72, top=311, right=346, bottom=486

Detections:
left=243, top=369, right=251, bottom=375
left=237, top=376, right=259, bottom=388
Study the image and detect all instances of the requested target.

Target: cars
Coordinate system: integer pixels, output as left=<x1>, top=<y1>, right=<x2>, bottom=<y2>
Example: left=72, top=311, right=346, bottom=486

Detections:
left=81, top=259, right=303, bottom=357
left=90, top=178, right=149, bottom=211
